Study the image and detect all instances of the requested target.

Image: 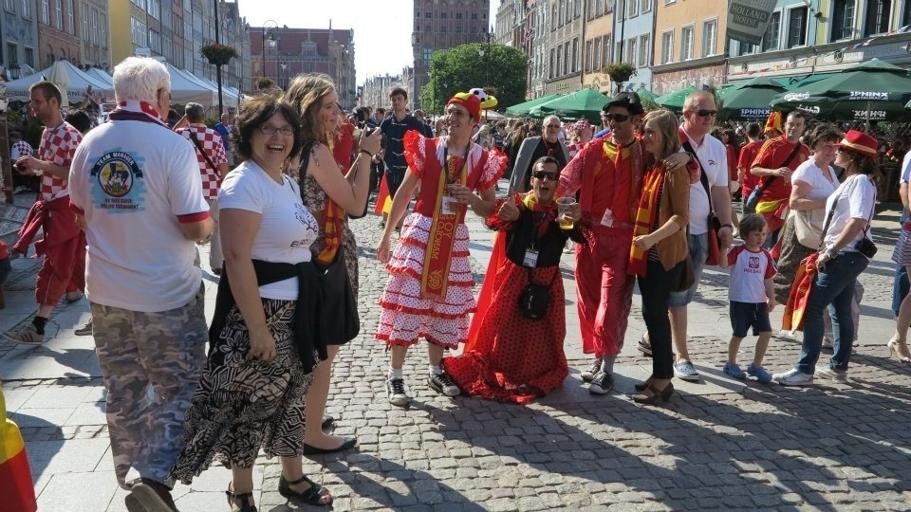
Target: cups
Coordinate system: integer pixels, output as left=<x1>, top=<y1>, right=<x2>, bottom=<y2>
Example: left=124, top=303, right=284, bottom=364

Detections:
left=783, top=172, right=792, bottom=187
left=448, top=184, right=460, bottom=203
left=556, top=196, right=577, bottom=230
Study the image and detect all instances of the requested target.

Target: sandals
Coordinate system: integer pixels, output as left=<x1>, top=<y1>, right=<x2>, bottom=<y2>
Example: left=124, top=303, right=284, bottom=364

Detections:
left=278, top=474, right=332, bottom=506
left=227, top=481, right=257, bottom=512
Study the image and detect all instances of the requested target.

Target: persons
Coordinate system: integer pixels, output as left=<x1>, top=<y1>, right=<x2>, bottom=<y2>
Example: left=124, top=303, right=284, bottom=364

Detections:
left=377, top=91, right=508, bottom=407
left=3, top=81, right=92, bottom=344
left=627, top=109, right=695, bottom=404
left=282, top=72, right=382, bottom=456
left=170, top=95, right=333, bottom=512
left=720, top=213, right=778, bottom=384
left=441, top=156, right=583, bottom=406
left=635, top=90, right=734, bottom=380
left=11, top=77, right=910, bottom=363
left=773, top=128, right=879, bottom=387
left=68, top=57, right=215, bottom=512
left=552, top=91, right=701, bottom=396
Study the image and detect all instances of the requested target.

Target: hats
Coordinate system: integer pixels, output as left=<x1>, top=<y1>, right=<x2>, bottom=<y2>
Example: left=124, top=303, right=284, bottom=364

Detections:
left=602, top=92, right=645, bottom=116
left=832, top=129, right=878, bottom=157
left=447, top=88, right=497, bottom=122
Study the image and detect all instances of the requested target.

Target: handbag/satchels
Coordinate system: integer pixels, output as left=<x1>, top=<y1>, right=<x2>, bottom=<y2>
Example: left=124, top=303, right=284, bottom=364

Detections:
left=313, top=243, right=360, bottom=346
left=705, top=215, right=722, bottom=266
left=745, top=186, right=762, bottom=209
left=517, top=281, right=550, bottom=320
left=794, top=211, right=823, bottom=250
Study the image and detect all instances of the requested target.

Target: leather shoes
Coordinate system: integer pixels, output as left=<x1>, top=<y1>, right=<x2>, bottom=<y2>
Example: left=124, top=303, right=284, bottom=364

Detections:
left=322, top=416, right=334, bottom=429
left=302, top=435, right=357, bottom=455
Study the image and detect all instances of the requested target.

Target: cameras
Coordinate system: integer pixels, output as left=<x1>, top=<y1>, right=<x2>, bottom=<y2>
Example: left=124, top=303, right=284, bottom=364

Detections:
left=365, top=127, right=389, bottom=148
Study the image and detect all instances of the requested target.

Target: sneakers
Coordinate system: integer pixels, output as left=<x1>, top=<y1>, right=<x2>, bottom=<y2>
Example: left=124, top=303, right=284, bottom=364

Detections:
left=3, top=288, right=92, bottom=344
left=580, top=335, right=856, bottom=402
left=427, top=372, right=460, bottom=397
left=385, top=377, right=407, bottom=406
left=131, top=479, right=176, bottom=511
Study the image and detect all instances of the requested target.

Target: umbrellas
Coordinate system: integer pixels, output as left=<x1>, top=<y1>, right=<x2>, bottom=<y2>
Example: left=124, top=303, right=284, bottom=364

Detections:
left=768, top=58, right=911, bottom=134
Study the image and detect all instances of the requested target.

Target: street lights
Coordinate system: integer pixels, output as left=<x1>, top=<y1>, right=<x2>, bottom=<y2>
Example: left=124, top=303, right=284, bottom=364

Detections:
left=261, top=26, right=280, bottom=79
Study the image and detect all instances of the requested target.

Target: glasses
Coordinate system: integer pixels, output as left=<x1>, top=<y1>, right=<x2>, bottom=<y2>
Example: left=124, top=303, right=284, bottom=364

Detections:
left=532, top=170, right=558, bottom=182
left=603, top=113, right=632, bottom=122
left=257, top=123, right=293, bottom=136
left=690, top=110, right=718, bottom=117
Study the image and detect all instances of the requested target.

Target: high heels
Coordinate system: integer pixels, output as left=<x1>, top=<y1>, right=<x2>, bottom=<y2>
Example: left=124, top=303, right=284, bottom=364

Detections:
left=888, top=336, right=911, bottom=363
